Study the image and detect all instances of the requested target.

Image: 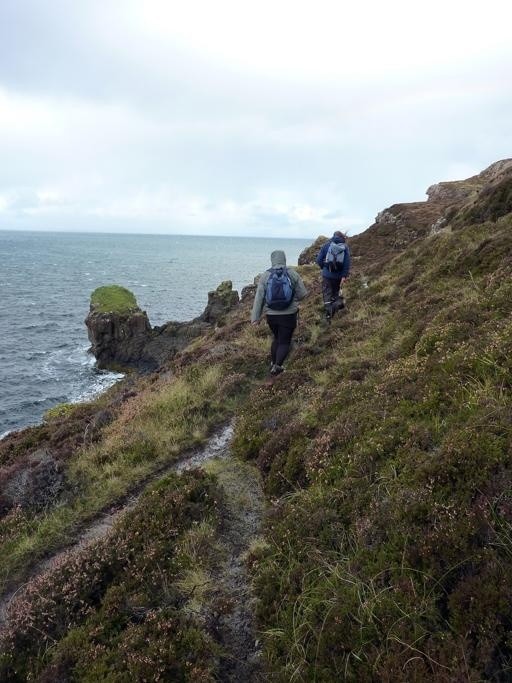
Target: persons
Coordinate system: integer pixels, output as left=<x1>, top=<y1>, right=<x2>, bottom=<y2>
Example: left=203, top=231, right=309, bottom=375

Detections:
left=317, top=230, right=352, bottom=318
left=250, top=249, right=308, bottom=374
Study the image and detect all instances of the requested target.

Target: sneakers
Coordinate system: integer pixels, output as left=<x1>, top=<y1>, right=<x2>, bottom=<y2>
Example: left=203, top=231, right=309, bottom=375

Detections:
left=269, top=361, right=284, bottom=376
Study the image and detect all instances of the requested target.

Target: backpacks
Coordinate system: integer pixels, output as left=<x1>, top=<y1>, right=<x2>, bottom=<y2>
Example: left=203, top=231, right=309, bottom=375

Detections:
left=266, top=267, right=295, bottom=311
left=322, top=241, right=348, bottom=273
